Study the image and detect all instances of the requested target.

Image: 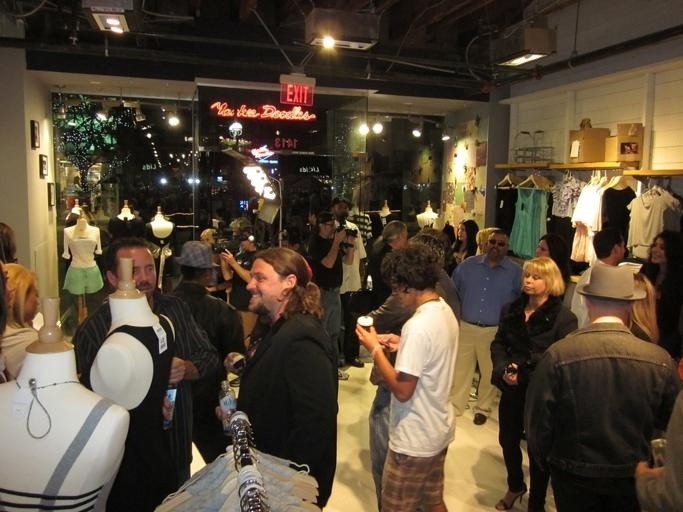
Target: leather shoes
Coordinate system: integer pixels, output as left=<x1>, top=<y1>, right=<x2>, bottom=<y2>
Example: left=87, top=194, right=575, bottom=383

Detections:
left=474, top=413, right=486, bottom=425
left=344, top=358, right=364, bottom=367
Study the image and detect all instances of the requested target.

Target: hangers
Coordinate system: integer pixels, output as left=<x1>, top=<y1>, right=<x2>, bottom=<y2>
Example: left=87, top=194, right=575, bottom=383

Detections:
left=156, top=410, right=322, bottom=512
left=494, top=167, right=680, bottom=202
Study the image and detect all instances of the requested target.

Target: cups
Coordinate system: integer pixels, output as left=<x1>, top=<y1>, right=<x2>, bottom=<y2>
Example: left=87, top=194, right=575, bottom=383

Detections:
left=650, top=438, right=667, bottom=468
left=358, top=317, right=375, bottom=333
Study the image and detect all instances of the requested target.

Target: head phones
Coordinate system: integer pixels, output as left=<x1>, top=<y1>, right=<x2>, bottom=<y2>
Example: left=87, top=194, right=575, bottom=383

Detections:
left=242, top=232, right=256, bottom=243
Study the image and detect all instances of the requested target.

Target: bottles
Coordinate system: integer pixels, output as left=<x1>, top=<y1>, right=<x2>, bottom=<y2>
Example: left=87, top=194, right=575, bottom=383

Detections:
left=218, top=380, right=240, bottom=438
left=162, top=383, right=180, bottom=433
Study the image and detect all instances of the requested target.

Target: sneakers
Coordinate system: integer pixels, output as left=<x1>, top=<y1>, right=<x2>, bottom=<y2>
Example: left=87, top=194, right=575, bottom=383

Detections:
left=230, top=376, right=241, bottom=387
left=337, top=369, right=349, bottom=380
left=468, top=392, right=479, bottom=402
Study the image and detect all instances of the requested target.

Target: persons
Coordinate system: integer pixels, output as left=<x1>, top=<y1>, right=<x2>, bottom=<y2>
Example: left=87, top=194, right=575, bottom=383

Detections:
left=1, top=195, right=682, bottom=512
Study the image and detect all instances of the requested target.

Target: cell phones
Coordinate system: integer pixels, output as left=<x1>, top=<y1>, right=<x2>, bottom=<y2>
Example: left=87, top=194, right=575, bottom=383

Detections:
left=378, top=339, right=390, bottom=350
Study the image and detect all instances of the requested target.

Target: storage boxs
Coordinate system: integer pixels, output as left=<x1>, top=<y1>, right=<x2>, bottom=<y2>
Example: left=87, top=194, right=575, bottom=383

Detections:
left=568, top=128, right=610, bottom=163
left=604, top=122, right=644, bottom=162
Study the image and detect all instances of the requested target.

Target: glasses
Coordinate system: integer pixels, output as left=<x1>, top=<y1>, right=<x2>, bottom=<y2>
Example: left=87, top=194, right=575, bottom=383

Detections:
left=325, top=222, right=334, bottom=228
left=489, top=239, right=505, bottom=246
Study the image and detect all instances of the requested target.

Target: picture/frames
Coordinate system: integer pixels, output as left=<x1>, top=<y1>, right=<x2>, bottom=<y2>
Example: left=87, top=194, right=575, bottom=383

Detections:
left=31, top=120, right=40, bottom=148
left=48, top=183, right=55, bottom=206
left=39, top=154, right=48, bottom=176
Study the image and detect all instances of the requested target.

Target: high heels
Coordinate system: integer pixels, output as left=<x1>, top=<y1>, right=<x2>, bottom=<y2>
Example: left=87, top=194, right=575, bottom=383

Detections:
left=495, top=482, right=527, bottom=510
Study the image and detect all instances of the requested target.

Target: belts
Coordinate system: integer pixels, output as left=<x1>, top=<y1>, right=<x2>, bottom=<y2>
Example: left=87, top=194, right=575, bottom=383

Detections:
left=467, top=321, right=496, bottom=327
left=322, top=287, right=339, bottom=294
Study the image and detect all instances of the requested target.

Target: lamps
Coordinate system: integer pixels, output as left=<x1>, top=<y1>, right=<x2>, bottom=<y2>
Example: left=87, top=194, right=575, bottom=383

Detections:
left=228, top=122, right=243, bottom=140
left=168, top=112, right=179, bottom=126
left=136, top=108, right=146, bottom=122
left=243, top=166, right=283, bottom=247
left=488, top=25, right=557, bottom=67
left=411, top=128, right=422, bottom=138
left=373, top=122, right=383, bottom=134
left=441, top=133, right=450, bottom=140
left=90, top=11, right=138, bottom=34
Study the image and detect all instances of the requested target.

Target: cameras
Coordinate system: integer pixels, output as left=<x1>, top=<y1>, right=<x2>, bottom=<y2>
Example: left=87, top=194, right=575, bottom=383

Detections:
left=212, top=221, right=233, bottom=238
left=211, top=239, right=241, bottom=253
left=335, top=216, right=358, bottom=237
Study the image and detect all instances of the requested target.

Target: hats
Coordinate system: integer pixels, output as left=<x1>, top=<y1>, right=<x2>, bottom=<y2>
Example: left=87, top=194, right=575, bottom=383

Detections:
left=233, top=226, right=258, bottom=241
left=316, top=212, right=337, bottom=225
left=576, top=263, right=647, bottom=301
left=174, top=240, right=221, bottom=269
left=326, top=197, right=353, bottom=212
